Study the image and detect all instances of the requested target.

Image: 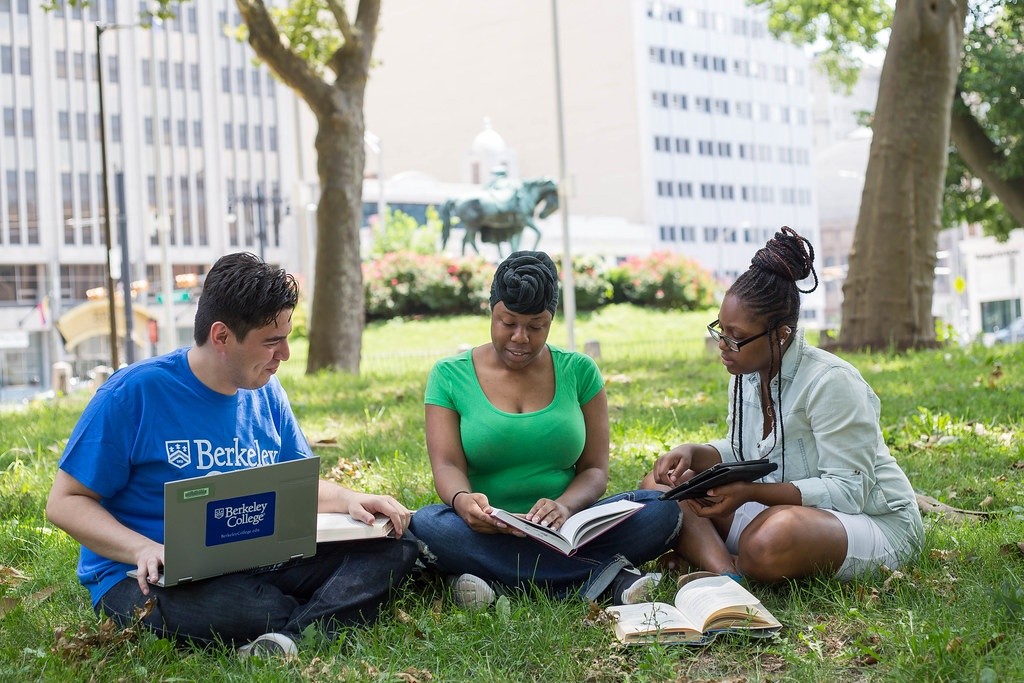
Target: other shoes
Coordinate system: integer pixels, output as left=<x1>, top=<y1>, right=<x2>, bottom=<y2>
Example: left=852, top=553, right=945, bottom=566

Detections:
left=610, top=565, right=662, bottom=606
left=236, top=632, right=298, bottom=669
left=677, top=571, right=741, bottom=589
left=454, top=573, right=495, bottom=609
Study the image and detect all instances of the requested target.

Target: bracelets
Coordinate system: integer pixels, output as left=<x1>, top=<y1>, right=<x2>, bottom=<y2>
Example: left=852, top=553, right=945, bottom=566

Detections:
left=452, top=490, right=471, bottom=513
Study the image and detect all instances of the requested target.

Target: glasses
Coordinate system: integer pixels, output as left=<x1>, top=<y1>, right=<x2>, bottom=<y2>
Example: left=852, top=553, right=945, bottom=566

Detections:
left=707, top=319, right=796, bottom=353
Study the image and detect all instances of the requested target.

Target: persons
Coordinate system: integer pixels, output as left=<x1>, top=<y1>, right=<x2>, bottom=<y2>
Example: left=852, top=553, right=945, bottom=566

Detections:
left=47, top=251, right=421, bottom=661
left=644, top=226, right=925, bottom=591
left=407, top=250, right=683, bottom=611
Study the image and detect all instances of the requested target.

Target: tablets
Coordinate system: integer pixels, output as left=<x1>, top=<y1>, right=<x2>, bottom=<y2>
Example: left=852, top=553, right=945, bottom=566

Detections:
left=658, top=459, right=770, bottom=501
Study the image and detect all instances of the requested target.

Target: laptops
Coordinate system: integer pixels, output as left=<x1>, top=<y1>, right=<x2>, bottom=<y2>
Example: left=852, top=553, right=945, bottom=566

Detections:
left=126, top=457, right=321, bottom=588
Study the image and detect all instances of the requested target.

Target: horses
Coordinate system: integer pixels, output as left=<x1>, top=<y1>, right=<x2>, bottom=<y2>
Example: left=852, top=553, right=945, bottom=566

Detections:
left=437, top=177, right=558, bottom=258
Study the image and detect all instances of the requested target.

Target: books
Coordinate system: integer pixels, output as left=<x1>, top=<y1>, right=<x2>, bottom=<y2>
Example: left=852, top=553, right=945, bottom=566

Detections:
left=489, top=500, right=646, bottom=560
left=605, top=575, right=783, bottom=648
left=317, top=509, right=416, bottom=541
